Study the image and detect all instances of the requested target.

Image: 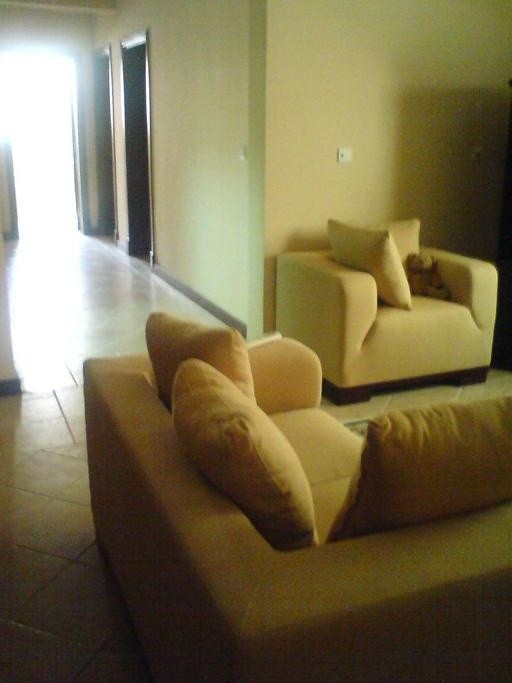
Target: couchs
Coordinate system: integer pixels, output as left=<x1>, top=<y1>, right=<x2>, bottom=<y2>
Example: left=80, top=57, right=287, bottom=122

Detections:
left=71, top=323, right=509, bottom=682
left=274, top=241, right=502, bottom=412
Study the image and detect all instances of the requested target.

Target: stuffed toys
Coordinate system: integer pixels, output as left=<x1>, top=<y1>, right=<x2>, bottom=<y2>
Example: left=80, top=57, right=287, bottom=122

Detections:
left=406, top=252, right=450, bottom=300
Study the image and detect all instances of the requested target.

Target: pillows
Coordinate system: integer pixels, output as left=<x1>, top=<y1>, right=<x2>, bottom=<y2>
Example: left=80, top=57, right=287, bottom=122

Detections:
left=330, top=392, right=510, bottom=539
left=321, top=217, right=411, bottom=306
left=143, top=312, right=257, bottom=418
left=354, top=218, right=437, bottom=267
left=172, top=355, right=320, bottom=548
